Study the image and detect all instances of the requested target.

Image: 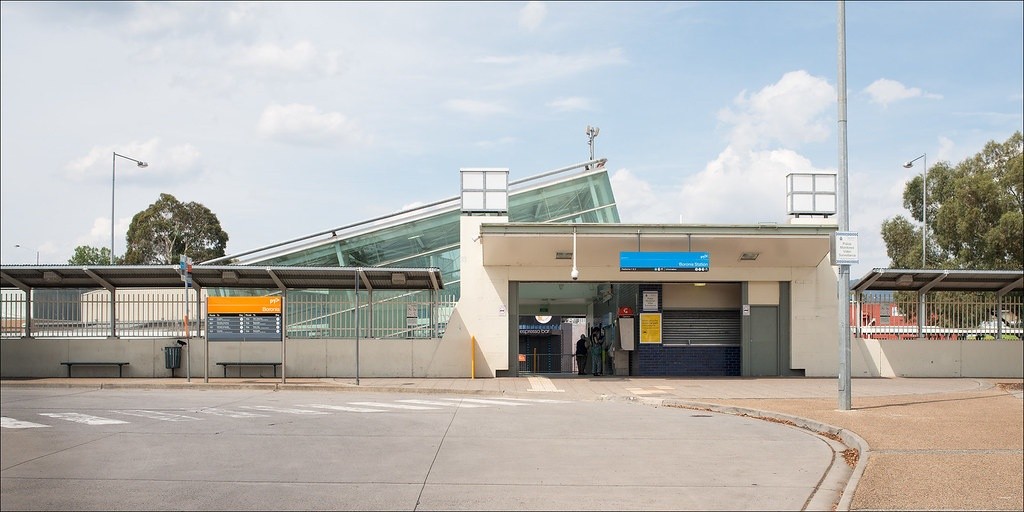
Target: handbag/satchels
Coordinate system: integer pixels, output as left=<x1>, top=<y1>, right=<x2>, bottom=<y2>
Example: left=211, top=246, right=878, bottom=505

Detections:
left=593, top=346, right=601, bottom=356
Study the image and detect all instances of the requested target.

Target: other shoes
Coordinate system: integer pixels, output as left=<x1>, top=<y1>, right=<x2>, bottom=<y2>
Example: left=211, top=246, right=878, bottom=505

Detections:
left=593, top=374, right=597, bottom=376
left=581, top=373, right=587, bottom=375
left=597, top=373, right=601, bottom=376
left=578, top=373, right=580, bottom=375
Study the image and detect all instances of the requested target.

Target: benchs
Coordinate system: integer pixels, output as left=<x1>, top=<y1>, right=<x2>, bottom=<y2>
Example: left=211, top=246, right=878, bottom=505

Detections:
left=216, top=363, right=282, bottom=377
left=61, top=362, right=130, bottom=377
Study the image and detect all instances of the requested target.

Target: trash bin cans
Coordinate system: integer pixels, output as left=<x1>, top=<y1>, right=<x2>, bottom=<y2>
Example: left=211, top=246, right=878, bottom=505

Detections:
left=165, top=347, right=181, bottom=369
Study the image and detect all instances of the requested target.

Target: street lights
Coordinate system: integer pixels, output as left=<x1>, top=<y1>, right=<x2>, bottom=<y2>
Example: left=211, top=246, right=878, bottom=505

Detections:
left=902, top=153, right=927, bottom=270
left=14, top=244, right=39, bottom=265
left=110, top=152, right=149, bottom=266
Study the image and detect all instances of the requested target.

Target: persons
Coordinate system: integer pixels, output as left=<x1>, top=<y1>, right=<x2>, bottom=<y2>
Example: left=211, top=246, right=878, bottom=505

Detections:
left=590, top=327, right=605, bottom=376
left=576, top=334, right=591, bottom=375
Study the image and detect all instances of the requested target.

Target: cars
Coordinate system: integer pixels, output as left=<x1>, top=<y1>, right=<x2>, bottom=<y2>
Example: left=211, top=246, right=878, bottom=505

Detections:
left=991, top=309, right=1022, bottom=328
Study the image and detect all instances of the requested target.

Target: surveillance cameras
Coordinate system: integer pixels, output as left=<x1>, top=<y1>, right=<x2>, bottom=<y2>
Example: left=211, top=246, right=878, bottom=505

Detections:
left=571, top=268, right=578, bottom=280
